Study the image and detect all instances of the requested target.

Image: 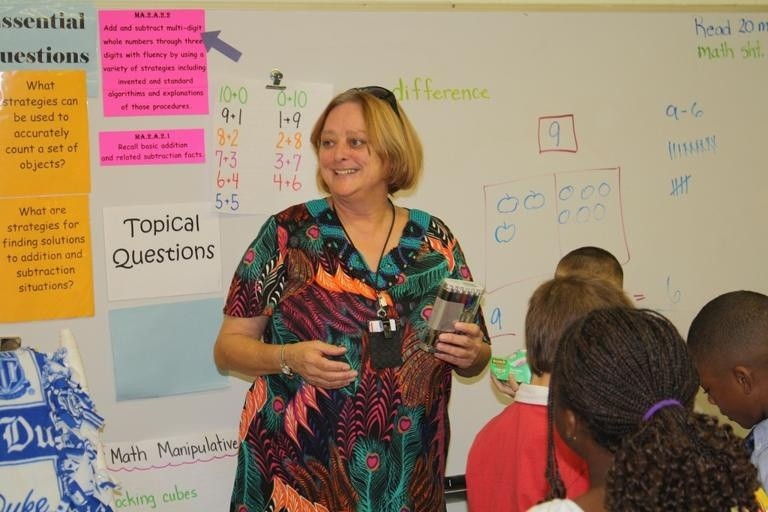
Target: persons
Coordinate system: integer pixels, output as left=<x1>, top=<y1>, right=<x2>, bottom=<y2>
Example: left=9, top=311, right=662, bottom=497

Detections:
left=211, top=87, right=492, bottom=510
left=466, top=275, right=636, bottom=512
left=682, top=284, right=767, bottom=490
left=520, top=306, right=759, bottom=512
left=487, top=245, right=625, bottom=399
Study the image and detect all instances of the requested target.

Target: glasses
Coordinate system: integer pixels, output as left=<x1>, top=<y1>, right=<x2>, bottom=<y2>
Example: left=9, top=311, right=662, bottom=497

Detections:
left=350, top=86, right=400, bottom=117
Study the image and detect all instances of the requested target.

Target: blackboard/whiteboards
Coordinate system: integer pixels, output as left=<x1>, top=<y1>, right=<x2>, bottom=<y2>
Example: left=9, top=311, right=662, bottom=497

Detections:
left=0, top=1, right=768, bottom=512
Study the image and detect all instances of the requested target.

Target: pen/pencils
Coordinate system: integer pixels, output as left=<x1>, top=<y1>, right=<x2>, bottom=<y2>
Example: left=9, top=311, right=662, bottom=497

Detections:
left=424, top=277, right=485, bottom=349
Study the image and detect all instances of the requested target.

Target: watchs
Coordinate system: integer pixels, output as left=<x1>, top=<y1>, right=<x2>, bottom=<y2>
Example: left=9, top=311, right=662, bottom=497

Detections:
left=278, top=344, right=291, bottom=375
